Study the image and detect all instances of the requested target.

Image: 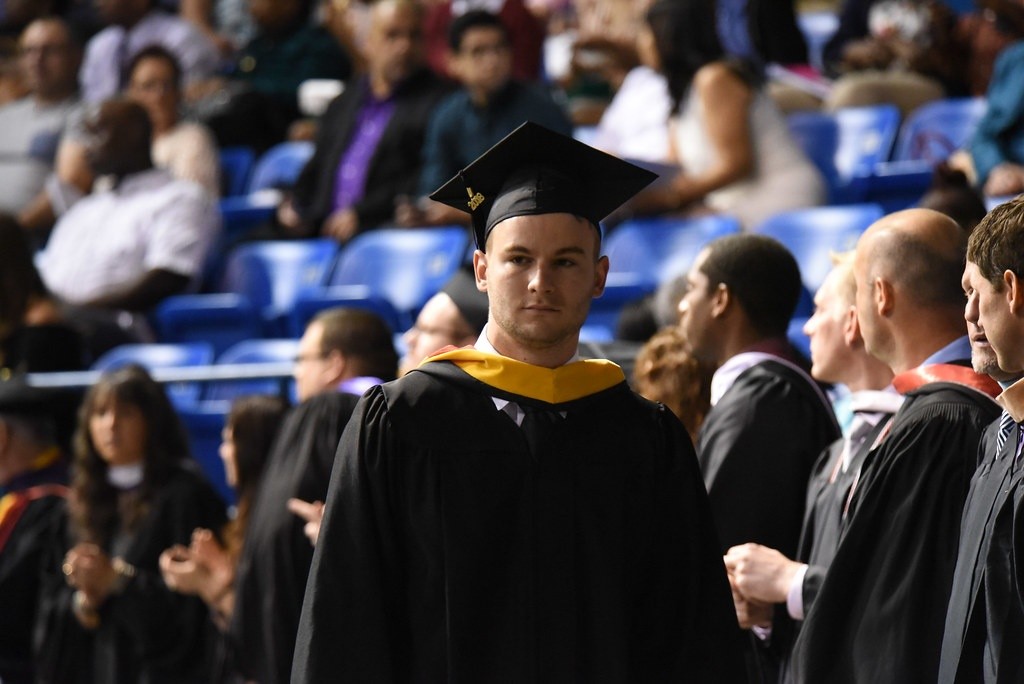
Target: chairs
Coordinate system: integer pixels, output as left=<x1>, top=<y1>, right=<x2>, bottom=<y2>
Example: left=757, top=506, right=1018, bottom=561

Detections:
left=24, top=98, right=1002, bottom=514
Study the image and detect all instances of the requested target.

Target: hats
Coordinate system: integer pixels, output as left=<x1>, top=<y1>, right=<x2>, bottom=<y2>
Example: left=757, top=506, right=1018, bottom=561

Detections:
left=1, top=366, right=44, bottom=409
left=429, top=123, right=660, bottom=253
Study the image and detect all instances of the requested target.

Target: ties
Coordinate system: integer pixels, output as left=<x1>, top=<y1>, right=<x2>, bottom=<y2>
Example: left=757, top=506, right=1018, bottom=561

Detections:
left=994, top=408, right=1016, bottom=462
left=842, top=415, right=870, bottom=474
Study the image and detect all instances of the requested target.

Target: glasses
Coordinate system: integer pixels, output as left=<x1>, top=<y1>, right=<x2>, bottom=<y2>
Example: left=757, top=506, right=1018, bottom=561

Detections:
left=294, top=354, right=320, bottom=365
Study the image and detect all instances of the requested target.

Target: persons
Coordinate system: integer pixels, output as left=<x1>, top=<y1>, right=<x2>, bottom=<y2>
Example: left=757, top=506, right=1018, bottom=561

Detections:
left=161, top=397, right=285, bottom=560
left=678, top=234, right=841, bottom=684
left=630, top=328, right=712, bottom=448
left=0, top=386, right=73, bottom=683
left=161, top=310, right=399, bottom=684
left=62, top=365, right=228, bottom=684
left=1, top=0, right=1024, bottom=371
left=292, top=123, right=745, bottom=683
left=943, top=198, right=1024, bottom=684
left=722, top=251, right=906, bottom=684
left=288, top=290, right=490, bottom=545
left=785, top=210, right=1002, bottom=684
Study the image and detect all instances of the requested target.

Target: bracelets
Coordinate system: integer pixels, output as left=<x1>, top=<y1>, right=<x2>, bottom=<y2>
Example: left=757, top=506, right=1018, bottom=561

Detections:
left=205, top=575, right=233, bottom=604
